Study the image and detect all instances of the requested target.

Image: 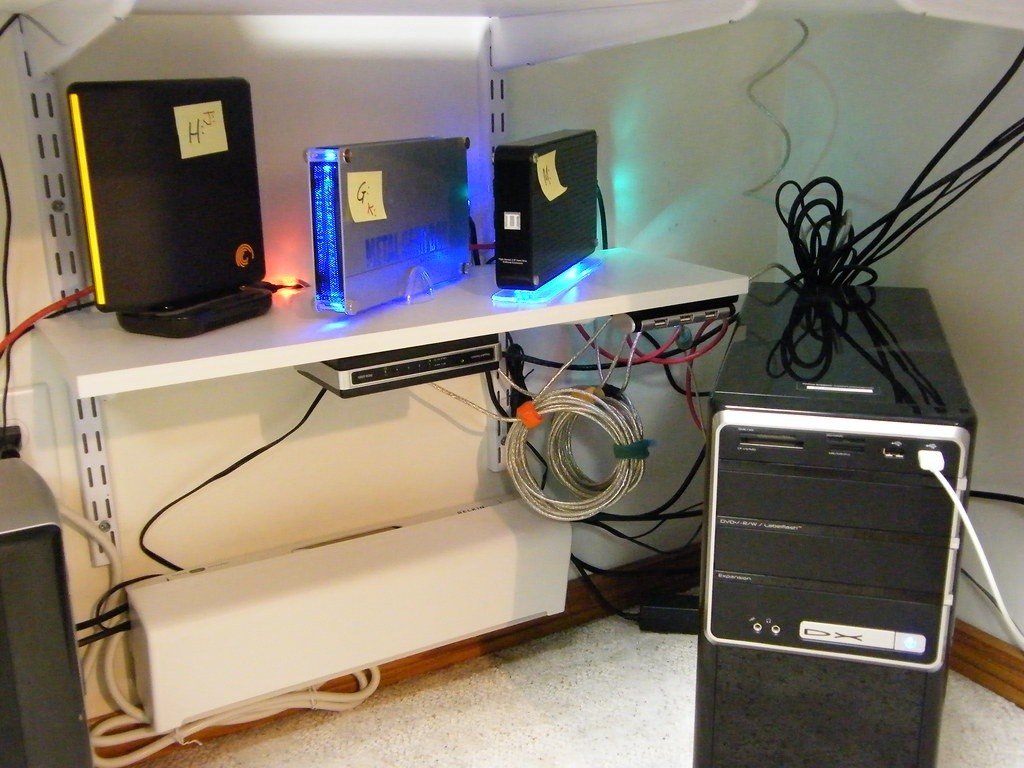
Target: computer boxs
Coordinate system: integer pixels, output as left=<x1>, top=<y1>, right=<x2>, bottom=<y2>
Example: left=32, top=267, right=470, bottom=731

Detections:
left=688, top=281, right=978, bottom=768
left=491, top=127, right=598, bottom=293
left=304, top=132, right=473, bottom=320
